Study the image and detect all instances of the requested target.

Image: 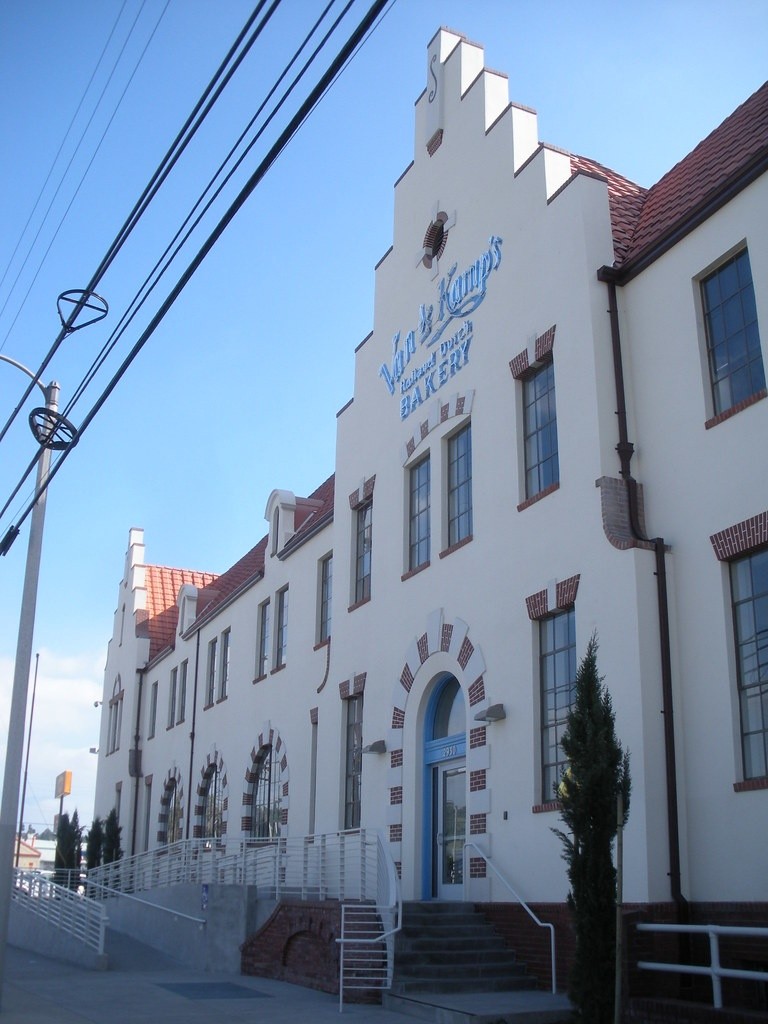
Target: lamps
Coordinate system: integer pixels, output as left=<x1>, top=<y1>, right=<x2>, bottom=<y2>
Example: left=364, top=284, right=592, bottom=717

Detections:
left=360, top=740, right=386, bottom=754
left=474, top=704, right=506, bottom=722
